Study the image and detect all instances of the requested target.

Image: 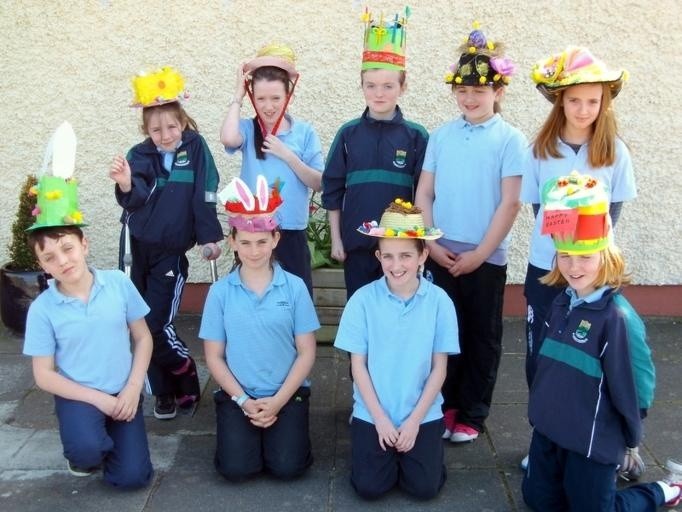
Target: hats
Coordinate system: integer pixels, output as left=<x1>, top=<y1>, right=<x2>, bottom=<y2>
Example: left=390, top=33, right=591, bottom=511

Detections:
left=360, top=5, right=409, bottom=72
left=531, top=46, right=628, bottom=105
left=215, top=175, right=286, bottom=233
left=445, top=20, right=513, bottom=88
left=127, top=66, right=191, bottom=108
left=241, top=45, right=299, bottom=80
left=23, top=175, right=90, bottom=234
left=542, top=171, right=612, bottom=255
left=355, top=199, right=444, bottom=241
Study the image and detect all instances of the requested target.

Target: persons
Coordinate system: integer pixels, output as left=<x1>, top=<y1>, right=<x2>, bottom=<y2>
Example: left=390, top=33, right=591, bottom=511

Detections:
left=217, top=62, right=327, bottom=301
left=236, top=392, right=251, bottom=407
left=522, top=245, right=682, bottom=512
left=197, top=226, right=323, bottom=485
left=332, top=238, right=461, bottom=499
left=19, top=224, right=158, bottom=491
left=516, top=47, right=648, bottom=482
left=106, top=103, right=224, bottom=423
left=408, top=83, right=531, bottom=449
left=320, top=68, right=432, bottom=428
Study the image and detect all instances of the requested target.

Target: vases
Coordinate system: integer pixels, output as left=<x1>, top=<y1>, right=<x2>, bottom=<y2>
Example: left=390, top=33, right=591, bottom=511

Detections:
left=309, top=267, right=347, bottom=344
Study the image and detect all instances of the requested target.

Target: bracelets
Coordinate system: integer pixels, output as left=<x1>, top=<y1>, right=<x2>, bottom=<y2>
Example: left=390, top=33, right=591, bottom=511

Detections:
left=226, top=98, right=242, bottom=108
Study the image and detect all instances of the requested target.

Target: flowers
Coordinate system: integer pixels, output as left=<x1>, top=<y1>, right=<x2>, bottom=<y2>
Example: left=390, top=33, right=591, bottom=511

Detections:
left=306, top=188, right=345, bottom=268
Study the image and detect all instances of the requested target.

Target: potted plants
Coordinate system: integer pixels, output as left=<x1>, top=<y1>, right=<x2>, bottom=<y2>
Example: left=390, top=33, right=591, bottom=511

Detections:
left=1, top=176, right=53, bottom=335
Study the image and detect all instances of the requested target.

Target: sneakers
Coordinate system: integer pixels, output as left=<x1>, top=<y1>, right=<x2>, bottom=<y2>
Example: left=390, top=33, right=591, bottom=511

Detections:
left=615, top=450, right=646, bottom=482
left=449, top=421, right=479, bottom=443
left=658, top=458, right=682, bottom=509
left=441, top=408, right=459, bottom=438
left=154, top=395, right=177, bottom=420
left=66, top=460, right=93, bottom=477
